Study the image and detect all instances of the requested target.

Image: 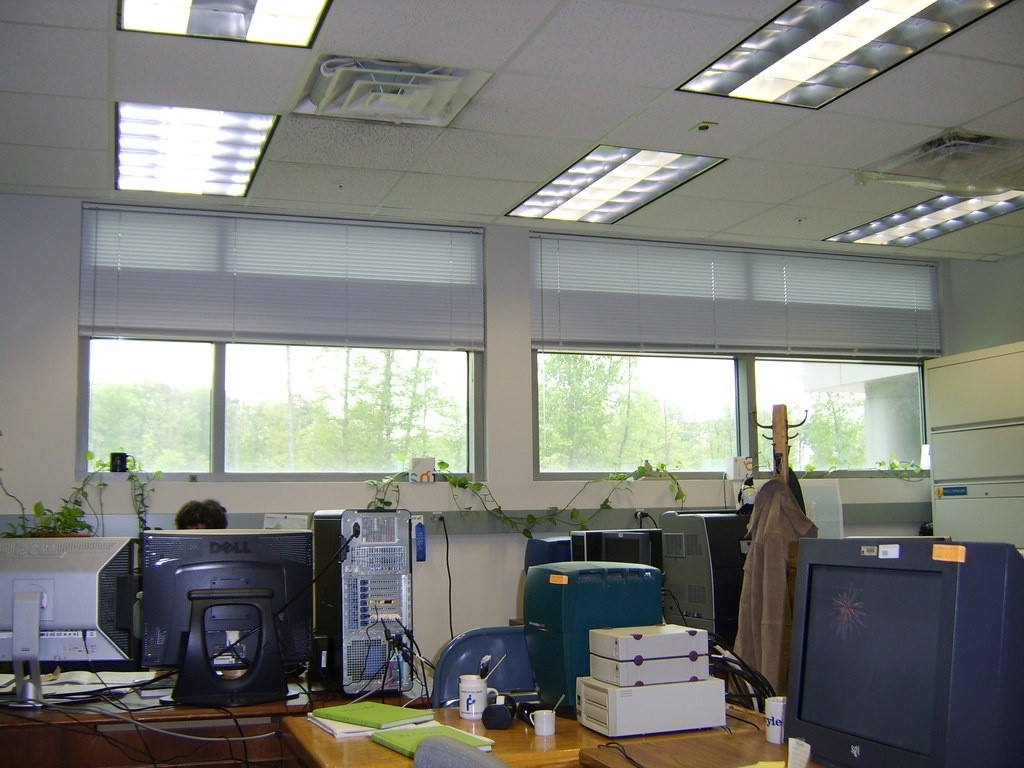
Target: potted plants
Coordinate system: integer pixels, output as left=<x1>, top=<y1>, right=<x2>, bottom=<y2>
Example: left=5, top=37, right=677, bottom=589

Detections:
left=11, top=453, right=163, bottom=538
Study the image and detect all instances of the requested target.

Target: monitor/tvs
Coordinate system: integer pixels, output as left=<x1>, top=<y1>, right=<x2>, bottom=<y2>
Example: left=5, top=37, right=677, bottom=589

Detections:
left=570, top=531, right=587, bottom=562
left=600, top=530, right=651, bottom=565
left=141, top=529, right=315, bottom=706
left=0, top=537, right=135, bottom=708
left=781, top=537, right=1024, bottom=768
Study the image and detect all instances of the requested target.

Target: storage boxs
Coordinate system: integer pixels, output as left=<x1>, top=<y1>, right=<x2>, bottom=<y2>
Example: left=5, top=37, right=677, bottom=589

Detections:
left=590, top=627, right=711, bottom=686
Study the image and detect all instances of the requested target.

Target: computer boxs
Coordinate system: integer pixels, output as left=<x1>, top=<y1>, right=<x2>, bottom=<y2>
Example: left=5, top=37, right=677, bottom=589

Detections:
left=524, top=560, right=662, bottom=717
left=314, top=507, right=415, bottom=695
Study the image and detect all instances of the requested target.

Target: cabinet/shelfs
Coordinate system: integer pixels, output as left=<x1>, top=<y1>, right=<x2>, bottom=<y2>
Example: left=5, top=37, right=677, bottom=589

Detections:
left=924, top=340, right=1024, bottom=559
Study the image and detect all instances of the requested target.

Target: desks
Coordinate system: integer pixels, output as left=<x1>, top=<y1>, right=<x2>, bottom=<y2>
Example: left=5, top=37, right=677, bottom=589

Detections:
left=277, top=688, right=772, bottom=768
left=579, top=729, right=828, bottom=768
left=0, top=665, right=438, bottom=729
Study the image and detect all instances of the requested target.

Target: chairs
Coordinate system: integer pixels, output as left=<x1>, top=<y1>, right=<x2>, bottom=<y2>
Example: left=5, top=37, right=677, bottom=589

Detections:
left=432, top=625, right=535, bottom=709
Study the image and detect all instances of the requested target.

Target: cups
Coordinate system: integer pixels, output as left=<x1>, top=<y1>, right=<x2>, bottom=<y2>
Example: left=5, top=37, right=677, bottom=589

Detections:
left=529, top=710, right=556, bottom=736
left=110, top=453, right=135, bottom=472
left=458, top=675, right=498, bottom=720
left=765, top=697, right=787, bottom=744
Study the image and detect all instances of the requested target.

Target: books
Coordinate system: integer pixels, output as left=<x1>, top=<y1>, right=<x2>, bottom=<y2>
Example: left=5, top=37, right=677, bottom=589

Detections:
left=307, top=701, right=496, bottom=759
left=132, top=671, right=178, bottom=697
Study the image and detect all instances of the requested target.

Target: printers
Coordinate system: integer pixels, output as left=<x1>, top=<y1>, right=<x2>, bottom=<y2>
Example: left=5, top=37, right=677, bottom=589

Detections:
left=659, top=510, right=754, bottom=644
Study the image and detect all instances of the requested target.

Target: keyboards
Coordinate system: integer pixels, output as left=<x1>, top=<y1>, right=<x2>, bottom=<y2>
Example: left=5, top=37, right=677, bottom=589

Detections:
left=139, top=679, right=177, bottom=691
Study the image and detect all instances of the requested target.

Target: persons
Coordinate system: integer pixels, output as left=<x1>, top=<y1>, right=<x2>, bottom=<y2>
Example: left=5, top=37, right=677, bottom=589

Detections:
left=174, top=499, right=228, bottom=529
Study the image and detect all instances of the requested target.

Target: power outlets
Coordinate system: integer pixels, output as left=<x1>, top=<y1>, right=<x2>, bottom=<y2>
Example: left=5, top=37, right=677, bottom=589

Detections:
left=430, top=514, right=448, bottom=521
